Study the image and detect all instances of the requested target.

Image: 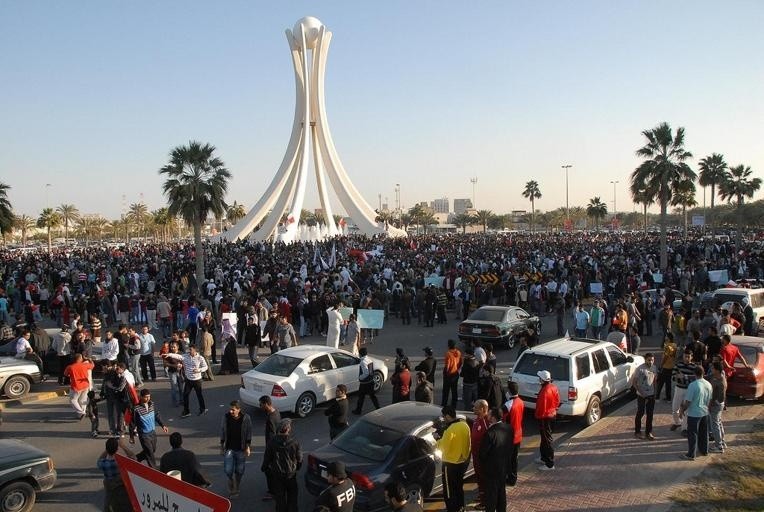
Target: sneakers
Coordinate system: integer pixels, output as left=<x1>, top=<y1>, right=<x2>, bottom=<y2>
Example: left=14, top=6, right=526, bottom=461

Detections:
left=300, top=318, right=448, bottom=337
left=227, top=486, right=242, bottom=497
left=472, top=457, right=554, bottom=512
left=634, top=426, right=728, bottom=460
left=143, top=378, right=159, bottom=382
left=197, top=410, right=208, bottom=417
left=179, top=410, right=192, bottom=418
left=71, top=398, right=183, bottom=442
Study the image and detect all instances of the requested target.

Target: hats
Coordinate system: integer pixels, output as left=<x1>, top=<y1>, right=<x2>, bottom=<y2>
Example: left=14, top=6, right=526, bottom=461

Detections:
left=324, top=461, right=348, bottom=475
left=397, top=347, right=433, bottom=377
left=536, top=370, right=552, bottom=383
left=277, top=418, right=291, bottom=430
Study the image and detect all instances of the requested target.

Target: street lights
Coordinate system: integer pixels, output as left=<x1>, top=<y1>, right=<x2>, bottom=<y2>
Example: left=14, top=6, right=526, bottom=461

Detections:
left=396, top=182, right=402, bottom=211
left=561, top=164, right=573, bottom=220
left=610, top=181, right=620, bottom=219
left=470, top=176, right=478, bottom=209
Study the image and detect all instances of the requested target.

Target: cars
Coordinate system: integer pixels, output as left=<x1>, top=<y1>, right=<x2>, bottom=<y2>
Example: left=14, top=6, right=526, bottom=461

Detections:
left=456, top=304, right=542, bottom=352
left=301, top=399, right=477, bottom=512
left=591, top=223, right=684, bottom=237
left=637, top=276, right=764, bottom=405
left=236, top=341, right=390, bottom=423
left=0, top=354, right=42, bottom=401
left=0, top=433, right=60, bottom=511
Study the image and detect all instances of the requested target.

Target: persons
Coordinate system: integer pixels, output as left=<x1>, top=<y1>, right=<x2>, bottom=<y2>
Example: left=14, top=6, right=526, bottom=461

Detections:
left=0, top=221, right=763, bottom=512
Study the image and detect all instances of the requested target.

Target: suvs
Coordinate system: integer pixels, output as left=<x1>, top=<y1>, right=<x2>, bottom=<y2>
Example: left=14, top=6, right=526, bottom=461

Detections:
left=501, top=334, right=648, bottom=431
